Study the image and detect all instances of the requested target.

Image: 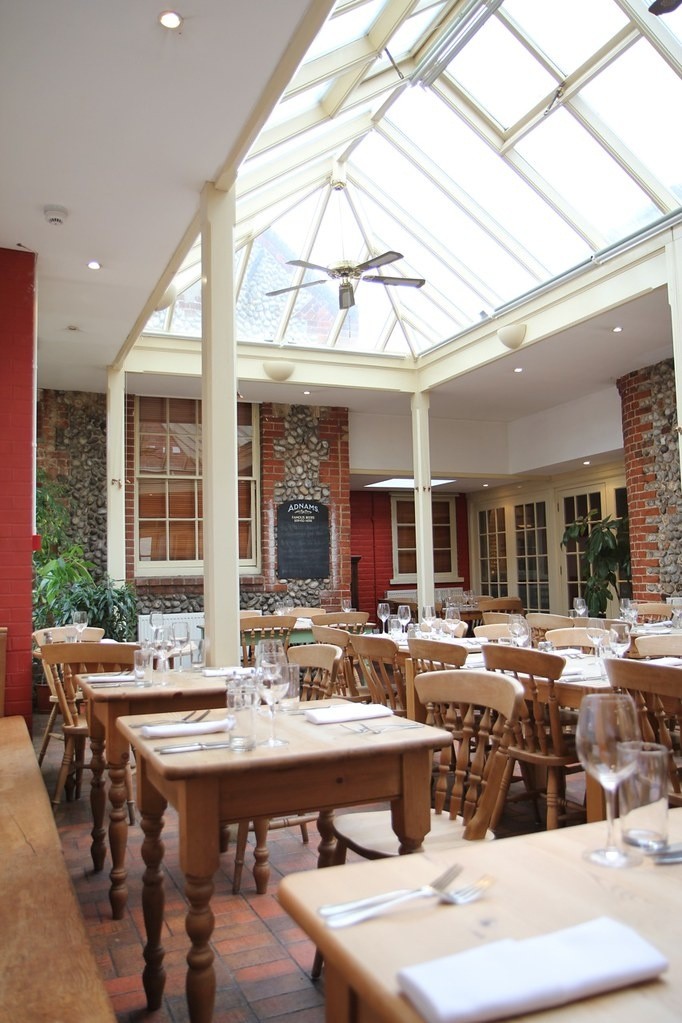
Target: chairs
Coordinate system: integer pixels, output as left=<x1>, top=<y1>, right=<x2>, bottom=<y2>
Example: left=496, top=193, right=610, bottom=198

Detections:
left=31, top=588, right=682, bottom=1023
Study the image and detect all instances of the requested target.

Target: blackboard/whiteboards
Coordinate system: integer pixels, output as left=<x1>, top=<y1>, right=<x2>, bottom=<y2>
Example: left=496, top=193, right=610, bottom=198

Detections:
left=277, top=499, right=330, bottom=579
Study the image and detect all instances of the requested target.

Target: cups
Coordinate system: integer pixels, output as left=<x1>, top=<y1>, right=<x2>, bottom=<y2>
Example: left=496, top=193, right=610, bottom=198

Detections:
left=392, top=620, right=403, bottom=635
left=227, top=674, right=257, bottom=751
left=134, top=650, right=152, bottom=686
left=582, top=606, right=589, bottom=618
left=600, top=632, right=613, bottom=658
left=432, top=618, right=442, bottom=637
left=277, top=663, right=302, bottom=711
left=350, top=608, right=357, bottom=612
left=616, top=740, right=669, bottom=850
left=387, top=614, right=399, bottom=635
left=65, top=624, right=78, bottom=643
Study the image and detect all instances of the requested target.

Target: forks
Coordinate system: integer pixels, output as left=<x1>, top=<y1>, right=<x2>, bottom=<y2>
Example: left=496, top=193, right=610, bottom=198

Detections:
left=340, top=721, right=424, bottom=733
left=318, top=864, right=495, bottom=930
left=129, top=709, right=211, bottom=727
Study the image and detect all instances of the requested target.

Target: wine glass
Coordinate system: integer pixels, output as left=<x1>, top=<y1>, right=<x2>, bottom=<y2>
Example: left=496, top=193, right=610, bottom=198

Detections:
left=275, top=599, right=295, bottom=617
left=586, top=618, right=606, bottom=657
left=573, top=597, right=586, bottom=617
left=620, top=597, right=640, bottom=629
left=341, top=599, right=350, bottom=611
left=73, top=611, right=89, bottom=642
left=253, top=640, right=289, bottom=747
left=608, top=623, right=632, bottom=659
left=148, top=611, right=191, bottom=685
left=378, top=590, right=529, bottom=647
left=575, top=693, right=642, bottom=867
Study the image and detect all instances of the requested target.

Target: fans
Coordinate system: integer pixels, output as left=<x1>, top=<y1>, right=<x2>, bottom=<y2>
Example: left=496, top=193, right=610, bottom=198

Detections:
left=264, top=186, right=425, bottom=311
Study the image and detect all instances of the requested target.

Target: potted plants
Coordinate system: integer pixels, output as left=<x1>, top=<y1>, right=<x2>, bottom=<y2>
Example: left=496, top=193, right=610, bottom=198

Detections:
left=33, top=468, right=139, bottom=714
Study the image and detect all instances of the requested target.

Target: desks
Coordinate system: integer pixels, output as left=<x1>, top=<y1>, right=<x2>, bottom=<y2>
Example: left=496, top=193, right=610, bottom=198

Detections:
left=196, top=611, right=377, bottom=655
left=72, top=660, right=269, bottom=919
left=417, top=600, right=482, bottom=626
left=277, top=806, right=682, bottom=1023
left=568, top=604, right=681, bottom=623
left=385, top=596, right=469, bottom=613
left=345, top=630, right=682, bottom=825
left=583, top=616, right=682, bottom=644
left=32, top=639, right=196, bottom=677
left=113, top=696, right=451, bottom=1023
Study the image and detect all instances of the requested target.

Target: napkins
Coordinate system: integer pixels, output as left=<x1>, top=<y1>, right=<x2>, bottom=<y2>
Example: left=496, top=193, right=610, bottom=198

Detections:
left=141, top=717, right=233, bottom=737
left=305, top=702, right=393, bottom=724
left=86, top=672, right=136, bottom=684
left=547, top=649, right=580, bottom=657
left=398, top=916, right=668, bottom=1023
left=199, top=666, right=256, bottom=676
left=644, top=657, right=681, bottom=666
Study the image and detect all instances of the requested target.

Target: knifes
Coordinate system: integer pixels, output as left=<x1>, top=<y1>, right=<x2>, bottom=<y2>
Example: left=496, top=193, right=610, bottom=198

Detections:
left=153, top=740, right=227, bottom=753
